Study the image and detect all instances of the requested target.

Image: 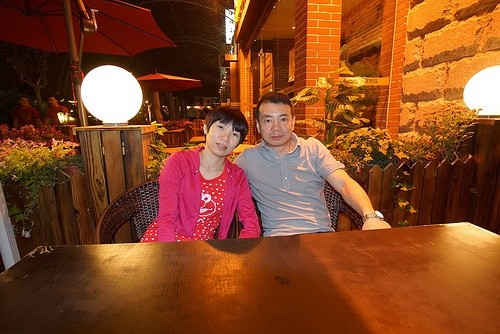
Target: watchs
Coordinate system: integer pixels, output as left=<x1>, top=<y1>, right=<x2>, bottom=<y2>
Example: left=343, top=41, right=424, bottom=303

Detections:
left=361, top=211, right=384, bottom=223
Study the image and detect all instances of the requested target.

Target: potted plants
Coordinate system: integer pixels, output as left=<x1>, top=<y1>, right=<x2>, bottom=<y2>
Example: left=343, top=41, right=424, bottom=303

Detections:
left=59, top=155, right=83, bottom=176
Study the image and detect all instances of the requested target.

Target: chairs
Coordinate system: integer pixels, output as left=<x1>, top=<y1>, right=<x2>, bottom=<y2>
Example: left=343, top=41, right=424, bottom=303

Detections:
left=95, top=180, right=363, bottom=244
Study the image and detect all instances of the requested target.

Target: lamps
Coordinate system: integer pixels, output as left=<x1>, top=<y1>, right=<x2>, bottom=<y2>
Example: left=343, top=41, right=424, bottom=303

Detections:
left=80, top=65, right=143, bottom=126
left=463, top=66, right=500, bottom=118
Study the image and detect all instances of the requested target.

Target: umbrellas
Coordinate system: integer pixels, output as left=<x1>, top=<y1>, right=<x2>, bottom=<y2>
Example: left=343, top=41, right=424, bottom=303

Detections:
left=134, top=71, right=202, bottom=113
left=0, top=0, right=174, bottom=125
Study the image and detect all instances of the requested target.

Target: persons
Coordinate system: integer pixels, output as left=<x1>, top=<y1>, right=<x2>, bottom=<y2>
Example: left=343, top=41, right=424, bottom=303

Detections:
left=232, top=91, right=391, bottom=238
left=140, top=107, right=260, bottom=243
left=11, top=95, right=42, bottom=129
left=143, top=105, right=210, bottom=122
left=44, top=94, right=70, bottom=126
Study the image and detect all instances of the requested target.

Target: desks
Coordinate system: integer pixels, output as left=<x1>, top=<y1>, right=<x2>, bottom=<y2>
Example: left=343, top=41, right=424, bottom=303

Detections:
left=189, top=137, right=249, bottom=143
left=0, top=222, right=500, bottom=334
left=163, top=144, right=255, bottom=155
